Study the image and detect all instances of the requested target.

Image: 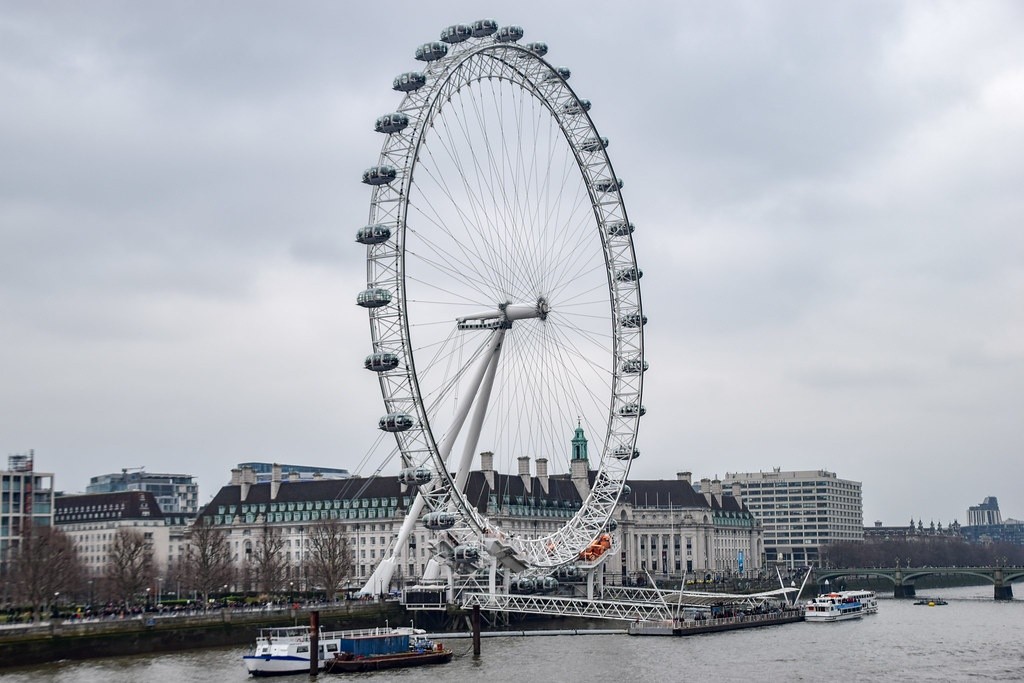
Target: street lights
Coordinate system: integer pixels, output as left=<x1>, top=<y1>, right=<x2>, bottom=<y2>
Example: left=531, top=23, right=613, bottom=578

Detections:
left=87, top=581, right=94, bottom=610
left=895, top=556, right=901, bottom=569
left=51, top=592, right=61, bottom=619
left=346, top=579, right=352, bottom=604
left=994, top=555, right=1001, bottom=567
left=298, top=525, right=304, bottom=594
left=379, top=576, right=385, bottom=599
left=223, top=584, right=229, bottom=607
left=354, top=523, right=360, bottom=588
left=289, top=581, right=295, bottom=603
left=145, top=587, right=150, bottom=612
left=1001, top=555, right=1008, bottom=567
left=905, top=557, right=912, bottom=568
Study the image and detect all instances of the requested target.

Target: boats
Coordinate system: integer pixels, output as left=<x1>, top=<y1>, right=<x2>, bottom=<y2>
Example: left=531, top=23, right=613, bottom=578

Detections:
left=804, top=594, right=862, bottom=622
left=913, top=598, right=948, bottom=606
left=834, top=588, right=879, bottom=613
left=241, top=624, right=426, bottom=678
left=323, top=636, right=452, bottom=671
